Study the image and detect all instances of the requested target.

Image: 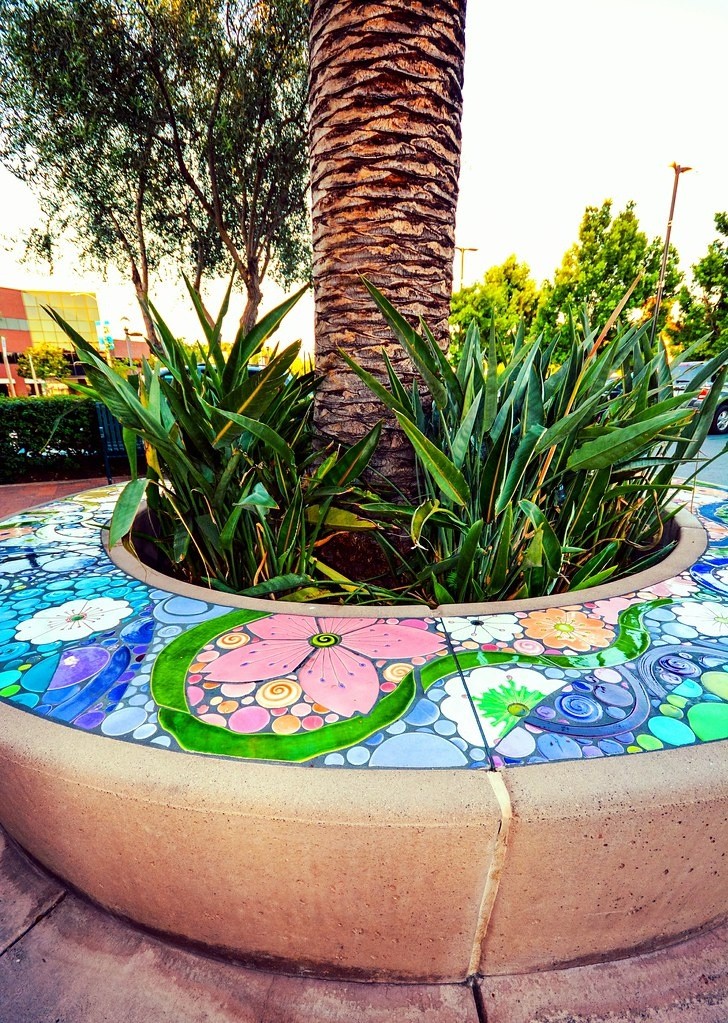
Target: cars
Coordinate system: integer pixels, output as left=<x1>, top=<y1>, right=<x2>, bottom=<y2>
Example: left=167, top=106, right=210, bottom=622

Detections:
left=607, top=359, right=728, bottom=434
left=151, top=363, right=297, bottom=389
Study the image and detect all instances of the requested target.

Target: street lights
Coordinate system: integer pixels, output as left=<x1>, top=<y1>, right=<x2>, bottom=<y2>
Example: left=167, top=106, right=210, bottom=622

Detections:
left=121, top=317, right=144, bottom=369
left=648, top=159, right=692, bottom=346
left=454, top=246, right=479, bottom=290
